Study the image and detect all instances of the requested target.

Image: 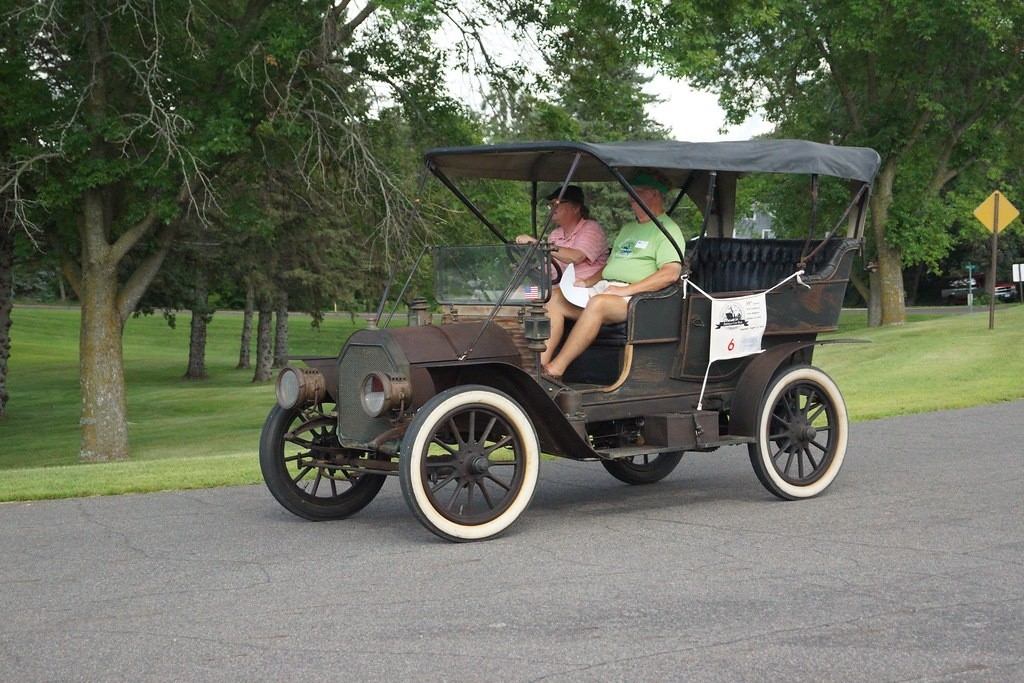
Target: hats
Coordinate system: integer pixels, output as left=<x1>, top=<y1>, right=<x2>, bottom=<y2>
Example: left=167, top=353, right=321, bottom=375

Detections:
left=629, top=168, right=673, bottom=194
left=545, top=185, right=585, bottom=205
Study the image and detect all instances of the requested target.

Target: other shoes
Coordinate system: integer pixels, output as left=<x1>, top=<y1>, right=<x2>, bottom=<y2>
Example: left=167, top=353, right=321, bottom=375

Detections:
left=540, top=366, right=563, bottom=384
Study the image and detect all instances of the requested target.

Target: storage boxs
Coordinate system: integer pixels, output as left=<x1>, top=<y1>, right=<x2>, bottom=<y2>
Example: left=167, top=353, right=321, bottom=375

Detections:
left=644, top=410, right=719, bottom=448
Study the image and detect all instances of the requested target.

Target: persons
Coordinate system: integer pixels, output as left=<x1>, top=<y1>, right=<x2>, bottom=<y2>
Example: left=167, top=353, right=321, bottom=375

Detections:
left=509, top=185, right=609, bottom=284
left=540, top=171, right=685, bottom=380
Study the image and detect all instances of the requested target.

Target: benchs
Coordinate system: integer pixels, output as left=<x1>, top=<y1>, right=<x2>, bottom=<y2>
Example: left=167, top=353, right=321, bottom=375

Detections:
left=564, top=248, right=693, bottom=337
left=691, top=237, right=861, bottom=293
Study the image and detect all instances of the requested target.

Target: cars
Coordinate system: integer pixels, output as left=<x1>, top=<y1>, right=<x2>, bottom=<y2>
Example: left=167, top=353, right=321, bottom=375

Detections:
left=941, top=275, right=1017, bottom=306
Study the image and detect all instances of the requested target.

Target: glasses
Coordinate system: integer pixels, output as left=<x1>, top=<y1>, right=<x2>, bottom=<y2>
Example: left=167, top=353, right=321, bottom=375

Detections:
left=545, top=200, right=568, bottom=209
